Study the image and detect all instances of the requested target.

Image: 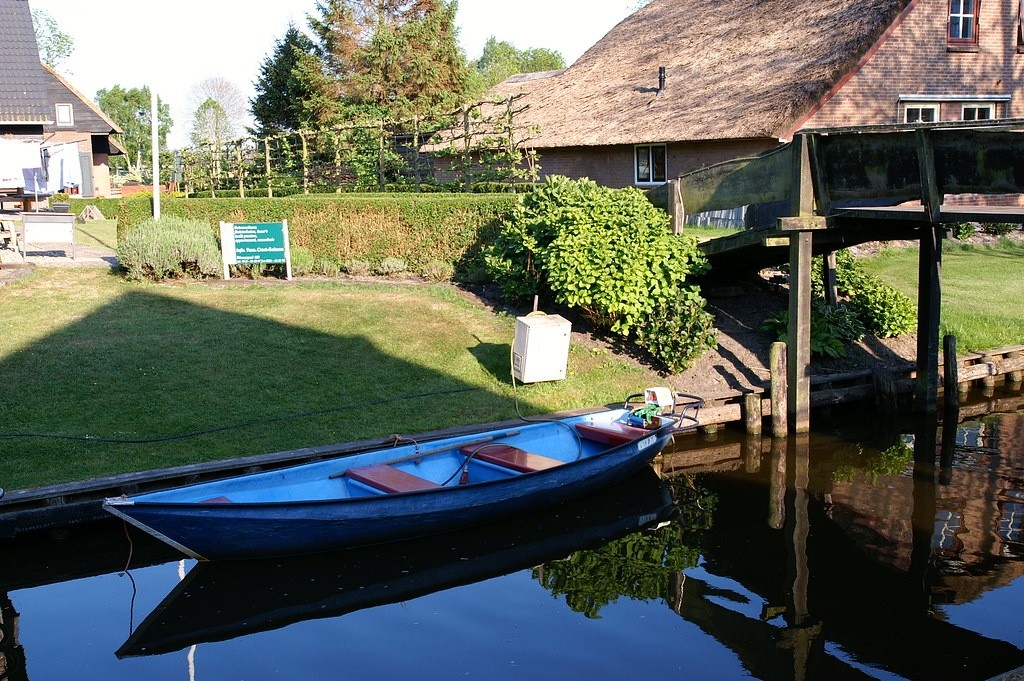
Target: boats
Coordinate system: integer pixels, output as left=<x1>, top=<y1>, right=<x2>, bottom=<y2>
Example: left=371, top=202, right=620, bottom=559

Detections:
left=115, top=463, right=701, bottom=662
left=101, top=386, right=707, bottom=563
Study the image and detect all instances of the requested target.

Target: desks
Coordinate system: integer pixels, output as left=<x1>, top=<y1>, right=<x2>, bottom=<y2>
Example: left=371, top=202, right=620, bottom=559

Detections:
left=0, top=196, right=46, bottom=214
left=20, top=212, right=76, bottom=260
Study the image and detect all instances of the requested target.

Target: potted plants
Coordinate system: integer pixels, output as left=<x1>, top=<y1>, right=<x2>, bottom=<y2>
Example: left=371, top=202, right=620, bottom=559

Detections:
left=53, top=193, right=70, bottom=213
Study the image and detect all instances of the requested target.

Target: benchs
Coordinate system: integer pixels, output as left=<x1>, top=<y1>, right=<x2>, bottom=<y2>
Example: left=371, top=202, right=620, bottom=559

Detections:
left=575, top=422, right=655, bottom=446
left=344, top=464, right=444, bottom=497
left=456, top=444, right=567, bottom=484
